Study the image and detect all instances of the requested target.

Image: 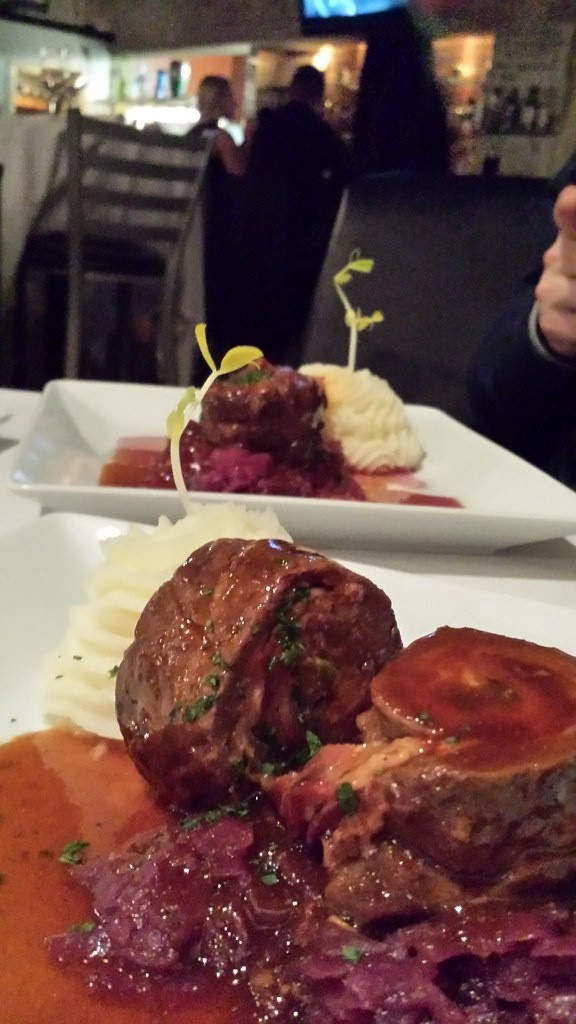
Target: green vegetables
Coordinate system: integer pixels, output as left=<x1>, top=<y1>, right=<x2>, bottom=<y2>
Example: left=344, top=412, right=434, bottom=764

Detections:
left=334, top=259, right=385, bottom=371
left=167, top=324, right=264, bottom=511
left=61, top=589, right=359, bottom=965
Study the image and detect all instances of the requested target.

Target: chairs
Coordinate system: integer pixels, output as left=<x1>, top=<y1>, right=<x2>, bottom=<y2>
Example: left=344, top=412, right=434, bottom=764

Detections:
left=20, top=106, right=219, bottom=386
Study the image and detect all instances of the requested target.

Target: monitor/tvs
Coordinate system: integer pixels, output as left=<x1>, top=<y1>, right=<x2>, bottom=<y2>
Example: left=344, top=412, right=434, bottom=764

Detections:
left=298, top=0, right=409, bottom=35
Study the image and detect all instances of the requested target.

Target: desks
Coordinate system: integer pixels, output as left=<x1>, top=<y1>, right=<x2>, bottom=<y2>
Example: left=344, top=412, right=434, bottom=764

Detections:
left=0, top=115, right=213, bottom=385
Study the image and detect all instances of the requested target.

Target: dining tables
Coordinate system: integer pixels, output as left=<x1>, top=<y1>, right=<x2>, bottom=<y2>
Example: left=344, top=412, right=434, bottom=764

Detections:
left=0, top=385, right=576, bottom=608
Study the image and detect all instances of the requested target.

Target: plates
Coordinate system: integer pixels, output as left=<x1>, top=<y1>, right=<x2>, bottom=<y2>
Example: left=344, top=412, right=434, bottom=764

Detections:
left=1, top=512, right=575, bottom=1024
left=7, top=379, right=576, bottom=555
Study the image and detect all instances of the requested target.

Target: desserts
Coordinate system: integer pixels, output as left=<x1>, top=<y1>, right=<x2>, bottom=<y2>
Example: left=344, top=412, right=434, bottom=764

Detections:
left=44, top=504, right=293, bottom=740
left=299, top=362, right=428, bottom=474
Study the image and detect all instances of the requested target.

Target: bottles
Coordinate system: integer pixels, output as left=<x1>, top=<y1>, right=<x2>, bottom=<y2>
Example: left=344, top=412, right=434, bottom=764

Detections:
left=480, top=84, right=548, bottom=136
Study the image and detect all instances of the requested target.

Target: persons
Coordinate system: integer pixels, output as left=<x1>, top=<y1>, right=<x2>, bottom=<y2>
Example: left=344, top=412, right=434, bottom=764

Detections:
left=466, top=151, right=576, bottom=492
left=183, top=67, right=356, bottom=378
